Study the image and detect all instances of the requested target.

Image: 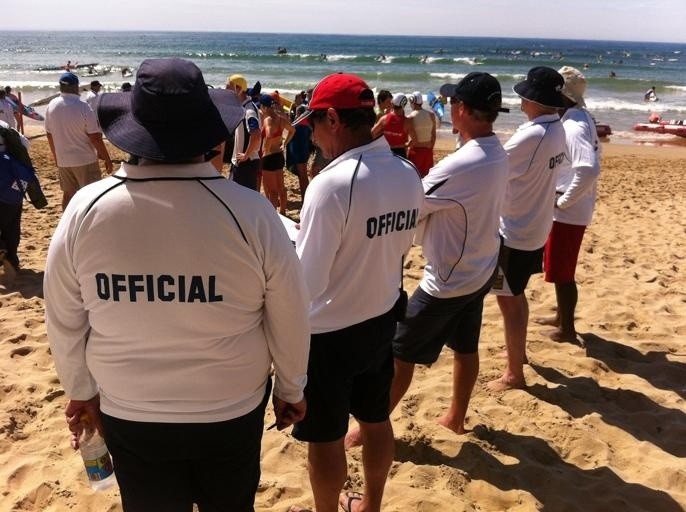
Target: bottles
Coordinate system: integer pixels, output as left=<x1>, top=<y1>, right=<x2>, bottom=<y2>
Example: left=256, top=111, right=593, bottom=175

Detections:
left=79, top=413, right=117, bottom=492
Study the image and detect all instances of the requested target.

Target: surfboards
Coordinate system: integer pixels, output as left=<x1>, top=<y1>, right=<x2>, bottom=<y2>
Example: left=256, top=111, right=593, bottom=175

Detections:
left=427, top=91, right=444, bottom=120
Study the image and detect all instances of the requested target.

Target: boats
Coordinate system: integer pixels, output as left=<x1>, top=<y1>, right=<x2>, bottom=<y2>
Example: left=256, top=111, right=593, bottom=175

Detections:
left=634, top=119, right=686, bottom=138
left=594, top=119, right=613, bottom=138
left=123, top=69, right=135, bottom=78
left=39, top=62, right=98, bottom=71
left=278, top=47, right=288, bottom=54
left=80, top=63, right=115, bottom=79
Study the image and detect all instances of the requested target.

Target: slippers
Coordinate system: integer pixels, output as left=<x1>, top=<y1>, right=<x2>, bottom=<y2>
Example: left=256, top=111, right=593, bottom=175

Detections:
left=340, top=492, right=363, bottom=512
left=286, top=506, right=312, bottom=512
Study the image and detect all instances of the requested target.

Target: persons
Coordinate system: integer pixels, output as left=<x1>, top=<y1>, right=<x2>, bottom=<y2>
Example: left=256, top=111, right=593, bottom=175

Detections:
left=294, top=75, right=423, bottom=512
left=534, top=67, right=602, bottom=341
left=344, top=71, right=509, bottom=447
left=644, top=87, right=655, bottom=100
left=0, top=61, right=133, bottom=273
left=44, top=58, right=311, bottom=512
left=481, top=67, right=577, bottom=390
left=371, top=89, right=467, bottom=179
left=222, top=76, right=325, bottom=229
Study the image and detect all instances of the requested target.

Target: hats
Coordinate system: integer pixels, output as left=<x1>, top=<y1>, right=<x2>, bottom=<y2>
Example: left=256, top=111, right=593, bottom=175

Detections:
left=59, top=73, right=78, bottom=86
left=558, top=66, right=587, bottom=108
left=290, top=72, right=375, bottom=127
left=122, top=83, right=131, bottom=89
left=409, top=91, right=422, bottom=105
left=228, top=74, right=279, bottom=108
left=440, top=72, right=509, bottom=113
left=91, top=81, right=102, bottom=86
left=97, top=59, right=246, bottom=161
left=391, top=93, right=407, bottom=108
left=512, top=67, right=577, bottom=110
